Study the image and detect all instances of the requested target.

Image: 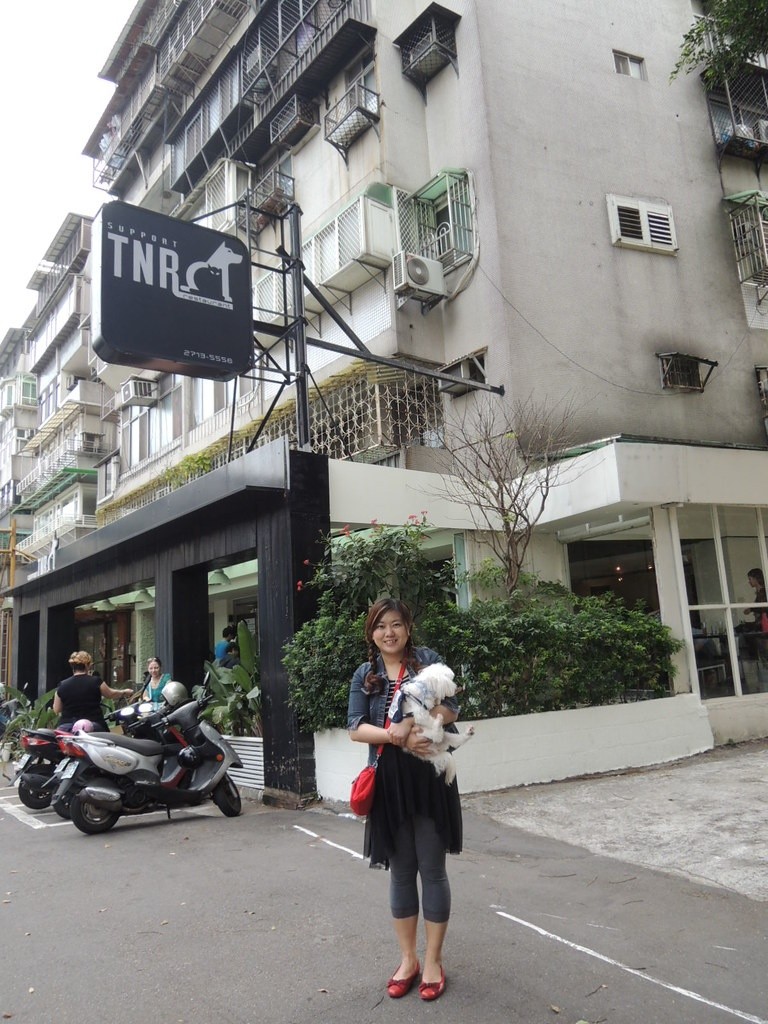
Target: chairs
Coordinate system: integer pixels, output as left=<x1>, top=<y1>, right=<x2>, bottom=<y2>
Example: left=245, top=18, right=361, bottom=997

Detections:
left=728, top=620, right=760, bottom=679
left=695, top=645, right=726, bottom=690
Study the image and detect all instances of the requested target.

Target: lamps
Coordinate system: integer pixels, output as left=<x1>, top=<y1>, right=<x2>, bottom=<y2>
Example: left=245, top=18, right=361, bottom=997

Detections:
left=133, top=588, right=155, bottom=603
left=96, top=599, right=117, bottom=611
left=207, top=568, right=232, bottom=586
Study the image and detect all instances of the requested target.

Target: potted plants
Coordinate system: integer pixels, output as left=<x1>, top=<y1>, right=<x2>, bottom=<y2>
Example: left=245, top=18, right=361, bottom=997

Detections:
left=250, top=201, right=271, bottom=225
left=262, top=186, right=283, bottom=210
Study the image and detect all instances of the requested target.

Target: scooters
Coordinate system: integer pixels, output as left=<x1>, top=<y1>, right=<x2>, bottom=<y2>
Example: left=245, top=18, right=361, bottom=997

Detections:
left=50, top=672, right=243, bottom=834
left=8, top=674, right=183, bottom=819
left=0, top=682, right=32, bottom=740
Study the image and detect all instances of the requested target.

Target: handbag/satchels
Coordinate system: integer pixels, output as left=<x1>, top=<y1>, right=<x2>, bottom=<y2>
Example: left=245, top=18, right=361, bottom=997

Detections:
left=351, top=760, right=378, bottom=815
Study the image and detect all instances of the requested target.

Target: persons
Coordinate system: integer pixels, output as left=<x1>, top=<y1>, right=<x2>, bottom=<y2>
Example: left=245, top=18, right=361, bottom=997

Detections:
left=219, top=642, right=240, bottom=669
left=215, top=625, right=237, bottom=665
left=142, top=670, right=150, bottom=683
left=92, top=670, right=100, bottom=676
left=141, top=657, right=172, bottom=710
left=743, top=567, right=768, bottom=672
left=0, top=683, right=7, bottom=709
left=53, top=650, right=134, bottom=734
left=346, top=597, right=464, bottom=1000
left=689, top=621, right=719, bottom=685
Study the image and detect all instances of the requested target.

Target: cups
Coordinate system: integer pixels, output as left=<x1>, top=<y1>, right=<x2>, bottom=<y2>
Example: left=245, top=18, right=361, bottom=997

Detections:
left=719, top=621, right=724, bottom=634
left=701, top=622, right=707, bottom=635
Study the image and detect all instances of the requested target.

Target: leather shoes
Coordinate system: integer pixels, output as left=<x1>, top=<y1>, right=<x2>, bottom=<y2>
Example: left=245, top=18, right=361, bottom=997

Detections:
left=386, top=959, right=420, bottom=998
left=418, top=964, right=445, bottom=1000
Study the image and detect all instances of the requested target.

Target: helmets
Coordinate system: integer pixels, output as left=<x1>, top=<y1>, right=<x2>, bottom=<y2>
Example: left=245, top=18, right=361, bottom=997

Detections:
left=177, top=745, right=203, bottom=769
left=160, top=681, right=189, bottom=707
left=72, top=719, right=93, bottom=735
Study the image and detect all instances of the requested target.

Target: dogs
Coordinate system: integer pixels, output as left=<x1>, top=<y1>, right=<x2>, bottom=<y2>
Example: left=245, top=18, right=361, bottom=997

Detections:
left=387, top=662, right=474, bottom=786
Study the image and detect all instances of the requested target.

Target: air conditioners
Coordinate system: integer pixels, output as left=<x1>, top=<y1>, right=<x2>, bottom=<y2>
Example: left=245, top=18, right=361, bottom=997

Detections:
left=121, top=381, right=158, bottom=406
left=393, top=254, right=445, bottom=301
left=410, top=32, right=447, bottom=75
left=736, top=224, right=762, bottom=251
left=245, top=45, right=277, bottom=76
left=277, top=103, right=311, bottom=143
left=440, top=361, right=478, bottom=395
left=66, top=374, right=88, bottom=389
left=752, top=119, right=768, bottom=152
